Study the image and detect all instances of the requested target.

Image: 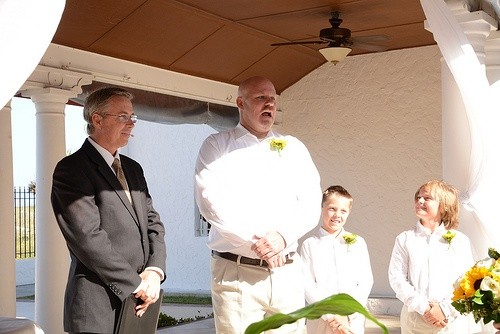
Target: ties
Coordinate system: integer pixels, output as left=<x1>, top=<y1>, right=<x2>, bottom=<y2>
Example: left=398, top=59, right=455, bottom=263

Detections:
left=111, top=158, right=135, bottom=210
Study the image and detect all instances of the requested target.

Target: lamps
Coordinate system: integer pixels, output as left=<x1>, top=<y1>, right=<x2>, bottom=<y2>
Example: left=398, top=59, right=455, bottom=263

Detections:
left=319, top=47, right=352, bottom=66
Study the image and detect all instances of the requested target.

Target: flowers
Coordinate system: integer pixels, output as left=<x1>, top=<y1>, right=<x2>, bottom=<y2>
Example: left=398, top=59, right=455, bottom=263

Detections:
left=451, top=245, right=500, bottom=333
left=442, top=230, right=457, bottom=244
left=270, top=137, right=287, bottom=153
left=342, top=233, right=359, bottom=245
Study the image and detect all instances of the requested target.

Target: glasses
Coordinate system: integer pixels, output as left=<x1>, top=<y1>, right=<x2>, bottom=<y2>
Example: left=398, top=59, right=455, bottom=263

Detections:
left=104, top=113, right=138, bottom=123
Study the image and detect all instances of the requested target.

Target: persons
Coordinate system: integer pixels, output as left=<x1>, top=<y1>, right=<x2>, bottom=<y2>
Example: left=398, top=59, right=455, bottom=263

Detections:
left=301, top=185, right=374, bottom=333
left=388, top=179, right=476, bottom=334
left=194, top=77, right=322, bottom=333
left=50, top=85, right=168, bottom=334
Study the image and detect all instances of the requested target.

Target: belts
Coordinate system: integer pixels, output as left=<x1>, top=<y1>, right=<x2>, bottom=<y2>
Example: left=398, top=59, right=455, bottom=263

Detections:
left=212, top=249, right=296, bottom=266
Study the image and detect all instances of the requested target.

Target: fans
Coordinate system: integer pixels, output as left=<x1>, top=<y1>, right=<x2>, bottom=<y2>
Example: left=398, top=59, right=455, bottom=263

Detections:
left=270, top=12, right=392, bottom=65
